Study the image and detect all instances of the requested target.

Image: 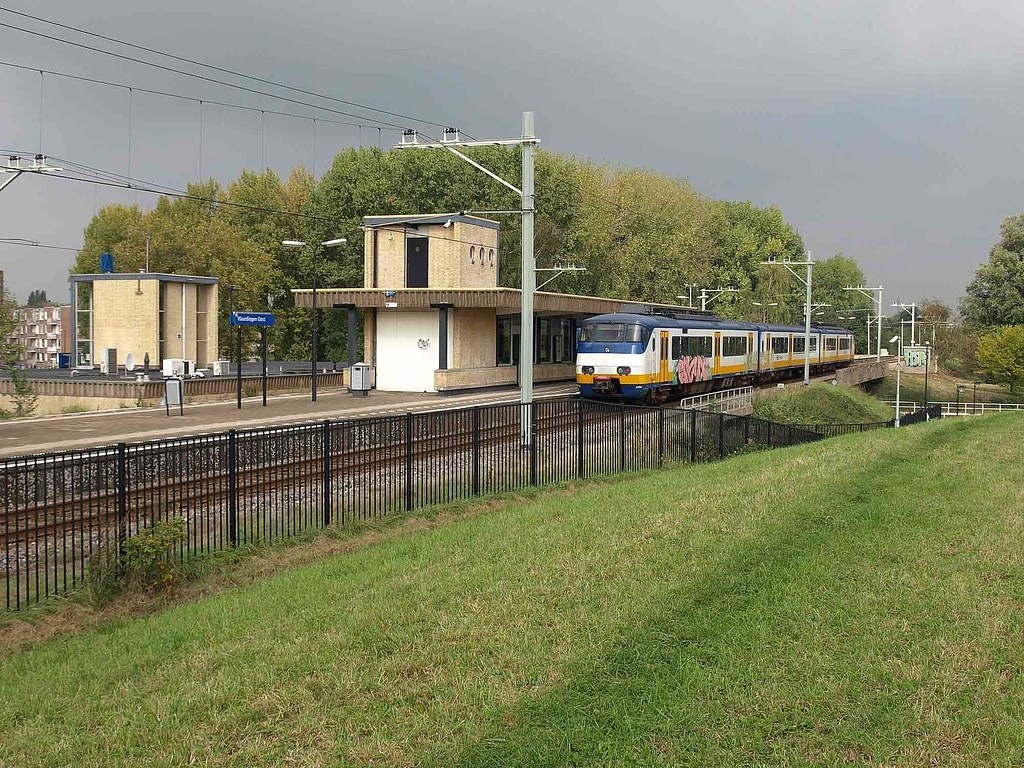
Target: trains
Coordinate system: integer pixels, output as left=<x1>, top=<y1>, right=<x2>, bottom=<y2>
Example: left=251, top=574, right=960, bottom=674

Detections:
left=571, top=310, right=856, bottom=406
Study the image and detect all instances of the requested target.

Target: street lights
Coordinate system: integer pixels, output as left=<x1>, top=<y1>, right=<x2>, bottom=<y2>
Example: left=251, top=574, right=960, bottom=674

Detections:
left=838, top=316, right=856, bottom=329
left=753, top=300, right=778, bottom=322
left=683, top=282, right=697, bottom=306
left=955, top=384, right=966, bottom=416
left=972, top=380, right=981, bottom=415
left=282, top=238, right=347, bottom=402
left=924, top=340, right=930, bottom=409
left=888, top=335, right=900, bottom=428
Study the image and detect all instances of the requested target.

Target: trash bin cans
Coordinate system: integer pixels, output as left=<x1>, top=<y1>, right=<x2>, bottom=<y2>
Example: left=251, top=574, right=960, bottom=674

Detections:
left=352, top=362, right=371, bottom=390
